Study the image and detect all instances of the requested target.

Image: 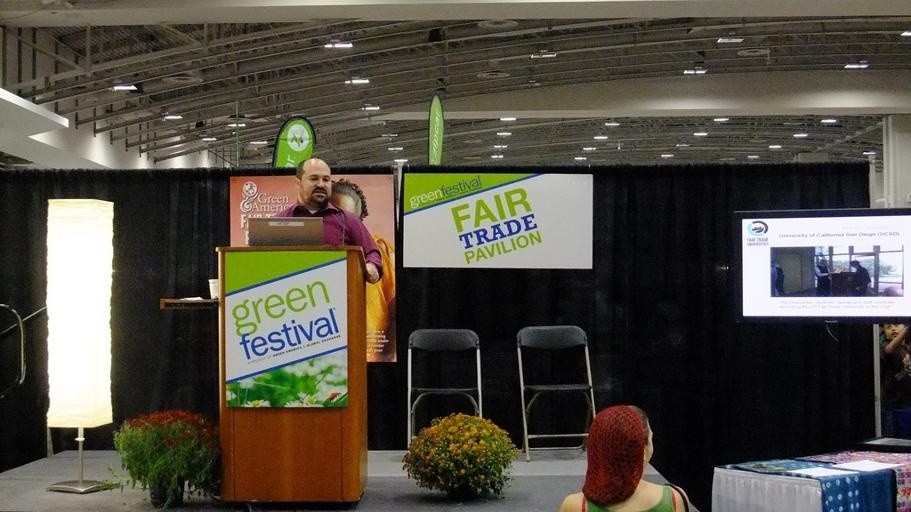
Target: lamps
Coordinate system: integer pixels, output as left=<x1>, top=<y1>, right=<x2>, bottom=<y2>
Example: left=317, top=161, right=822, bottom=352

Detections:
left=45, top=198, right=117, bottom=493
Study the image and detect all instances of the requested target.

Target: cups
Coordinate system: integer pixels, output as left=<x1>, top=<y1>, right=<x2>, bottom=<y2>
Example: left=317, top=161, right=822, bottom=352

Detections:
left=208, top=278, right=219, bottom=298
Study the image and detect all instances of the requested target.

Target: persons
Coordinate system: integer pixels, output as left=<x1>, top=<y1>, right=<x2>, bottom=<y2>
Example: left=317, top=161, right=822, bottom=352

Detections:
left=560, top=405, right=685, bottom=512
left=878, top=323, right=911, bottom=393
left=770, top=259, right=785, bottom=297
left=327, top=179, right=396, bottom=361
left=272, top=157, right=383, bottom=285
left=814, top=258, right=832, bottom=296
left=851, top=260, right=871, bottom=296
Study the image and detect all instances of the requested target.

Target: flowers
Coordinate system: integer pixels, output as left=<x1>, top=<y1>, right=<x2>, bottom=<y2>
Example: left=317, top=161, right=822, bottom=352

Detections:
left=97, top=411, right=222, bottom=511
left=401, top=412, right=519, bottom=501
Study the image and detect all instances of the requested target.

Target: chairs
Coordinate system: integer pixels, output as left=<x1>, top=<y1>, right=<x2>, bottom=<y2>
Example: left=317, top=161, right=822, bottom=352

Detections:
left=517, top=326, right=596, bottom=463
left=407, top=327, right=483, bottom=452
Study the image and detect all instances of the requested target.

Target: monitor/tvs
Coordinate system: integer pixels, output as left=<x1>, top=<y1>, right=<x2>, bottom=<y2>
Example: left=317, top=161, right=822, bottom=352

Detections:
left=248, top=216, right=325, bottom=248
left=728, top=205, right=911, bottom=327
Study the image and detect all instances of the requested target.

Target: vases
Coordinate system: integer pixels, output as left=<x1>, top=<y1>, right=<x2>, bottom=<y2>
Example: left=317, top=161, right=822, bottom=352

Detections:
left=147, top=474, right=185, bottom=508
left=444, top=487, right=479, bottom=502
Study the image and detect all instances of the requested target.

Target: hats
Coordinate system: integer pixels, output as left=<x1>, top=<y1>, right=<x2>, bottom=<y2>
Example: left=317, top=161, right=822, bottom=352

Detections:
left=582, top=405, right=645, bottom=508
